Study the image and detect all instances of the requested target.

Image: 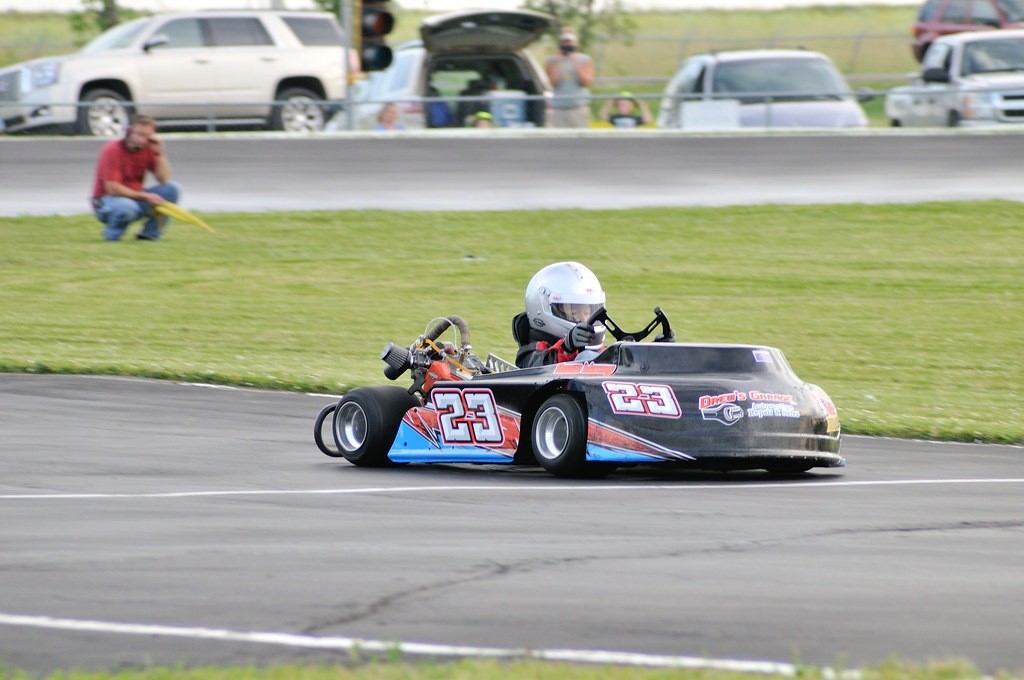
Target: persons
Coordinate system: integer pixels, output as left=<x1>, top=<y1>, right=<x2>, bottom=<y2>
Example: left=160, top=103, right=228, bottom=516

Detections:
left=470, top=110, right=494, bottom=129
left=511, top=258, right=607, bottom=394
left=548, top=26, right=596, bottom=127
left=606, top=90, right=646, bottom=128
left=93, top=114, right=181, bottom=244
left=369, top=102, right=405, bottom=131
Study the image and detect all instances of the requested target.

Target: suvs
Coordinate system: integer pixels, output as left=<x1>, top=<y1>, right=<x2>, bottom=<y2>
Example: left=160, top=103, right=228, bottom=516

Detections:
left=0, top=8, right=352, bottom=136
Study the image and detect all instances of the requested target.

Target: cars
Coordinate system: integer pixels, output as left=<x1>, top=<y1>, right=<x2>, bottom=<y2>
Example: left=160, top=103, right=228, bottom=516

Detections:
left=364, top=5, right=559, bottom=131
left=910, top=0, right=1024, bottom=61
left=656, top=50, right=877, bottom=130
left=890, top=29, right=1024, bottom=127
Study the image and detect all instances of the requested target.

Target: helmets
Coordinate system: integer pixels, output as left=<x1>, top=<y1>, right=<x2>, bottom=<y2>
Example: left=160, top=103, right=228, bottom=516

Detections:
left=613, top=90, right=632, bottom=100
left=524, top=260, right=608, bottom=350
left=473, top=110, right=492, bottom=121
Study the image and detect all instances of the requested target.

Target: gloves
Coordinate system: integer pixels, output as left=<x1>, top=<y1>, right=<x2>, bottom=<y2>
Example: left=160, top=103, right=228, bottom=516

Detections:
left=653, top=331, right=677, bottom=345
left=563, top=323, right=597, bottom=353
